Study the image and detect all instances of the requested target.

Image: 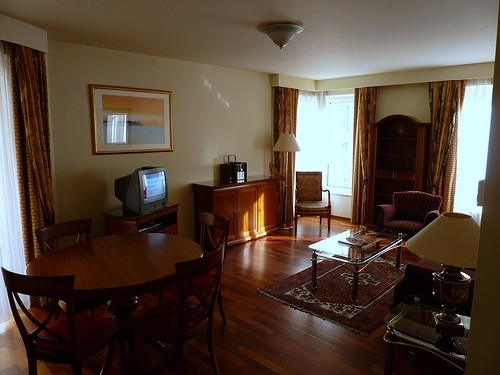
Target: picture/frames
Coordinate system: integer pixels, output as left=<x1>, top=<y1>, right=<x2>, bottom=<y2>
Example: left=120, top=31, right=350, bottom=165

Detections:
left=90, top=84, right=175, bottom=155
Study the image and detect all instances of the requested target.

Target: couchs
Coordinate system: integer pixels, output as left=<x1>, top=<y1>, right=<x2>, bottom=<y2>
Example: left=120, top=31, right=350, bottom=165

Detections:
left=373, top=190, right=443, bottom=239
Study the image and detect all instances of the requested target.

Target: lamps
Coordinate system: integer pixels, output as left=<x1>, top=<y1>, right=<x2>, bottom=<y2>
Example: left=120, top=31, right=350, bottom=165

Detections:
left=405, top=212, right=480, bottom=334
left=273, top=134, right=301, bottom=231
left=258, top=21, right=304, bottom=50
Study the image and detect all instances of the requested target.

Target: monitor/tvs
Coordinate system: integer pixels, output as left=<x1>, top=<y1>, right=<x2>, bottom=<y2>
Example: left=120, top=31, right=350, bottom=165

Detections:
left=114, top=166, right=168, bottom=216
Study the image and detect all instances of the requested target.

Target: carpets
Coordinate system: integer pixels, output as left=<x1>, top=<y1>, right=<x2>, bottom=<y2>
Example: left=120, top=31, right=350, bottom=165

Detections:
left=256, top=256, right=416, bottom=337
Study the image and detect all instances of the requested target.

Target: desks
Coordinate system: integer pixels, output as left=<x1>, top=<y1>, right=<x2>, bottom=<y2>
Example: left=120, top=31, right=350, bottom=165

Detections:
left=25, top=232, right=203, bottom=336
left=382, top=303, right=470, bottom=374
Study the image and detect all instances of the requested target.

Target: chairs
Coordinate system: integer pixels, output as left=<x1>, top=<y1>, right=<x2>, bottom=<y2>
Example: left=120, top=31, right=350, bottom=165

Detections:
left=35, top=218, right=92, bottom=261
left=159, top=210, right=227, bottom=324
left=296, top=171, right=331, bottom=233
left=128, top=246, right=223, bottom=375
left=2, top=267, right=120, bottom=375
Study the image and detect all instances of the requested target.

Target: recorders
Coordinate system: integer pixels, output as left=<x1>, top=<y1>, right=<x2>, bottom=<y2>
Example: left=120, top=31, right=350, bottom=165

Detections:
left=220, top=162, right=247, bottom=184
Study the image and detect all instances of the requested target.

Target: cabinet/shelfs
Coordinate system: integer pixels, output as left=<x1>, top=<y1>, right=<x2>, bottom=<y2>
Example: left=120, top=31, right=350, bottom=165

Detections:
left=364, top=113, right=431, bottom=225
left=193, top=175, right=284, bottom=249
left=103, top=202, right=180, bottom=241
